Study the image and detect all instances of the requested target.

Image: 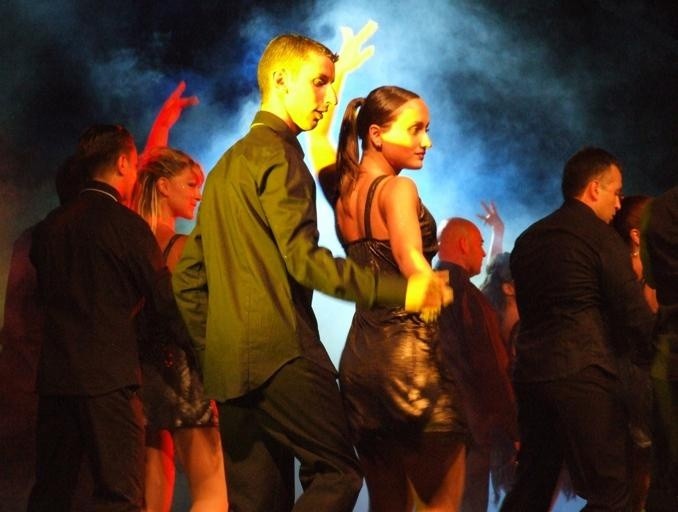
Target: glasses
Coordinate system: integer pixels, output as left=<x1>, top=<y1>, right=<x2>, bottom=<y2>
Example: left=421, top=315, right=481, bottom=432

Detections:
left=601, top=181, right=625, bottom=203
